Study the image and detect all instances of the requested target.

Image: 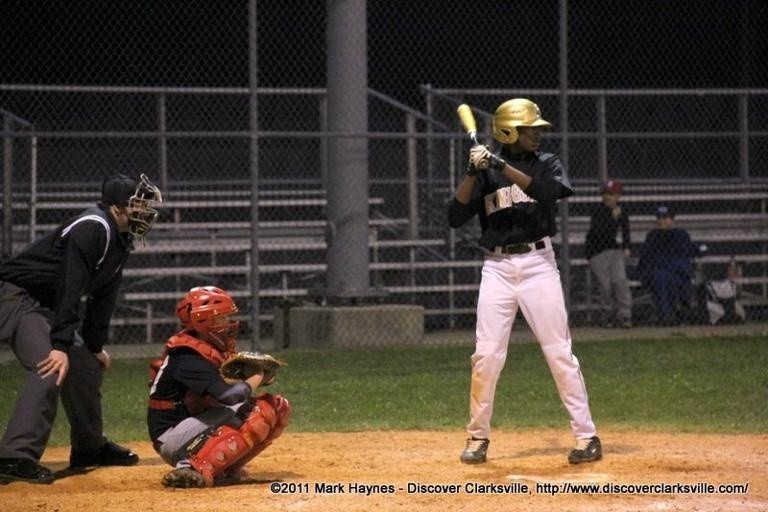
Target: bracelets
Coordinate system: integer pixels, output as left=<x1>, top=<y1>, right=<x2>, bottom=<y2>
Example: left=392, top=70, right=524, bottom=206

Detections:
left=497, top=159, right=506, bottom=172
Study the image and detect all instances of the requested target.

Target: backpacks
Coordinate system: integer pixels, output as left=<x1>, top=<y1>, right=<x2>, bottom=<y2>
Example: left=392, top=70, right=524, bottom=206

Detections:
left=704, top=280, right=746, bottom=326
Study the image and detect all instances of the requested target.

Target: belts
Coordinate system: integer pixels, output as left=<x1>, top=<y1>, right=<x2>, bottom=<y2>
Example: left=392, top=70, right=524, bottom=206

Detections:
left=488, top=241, right=545, bottom=254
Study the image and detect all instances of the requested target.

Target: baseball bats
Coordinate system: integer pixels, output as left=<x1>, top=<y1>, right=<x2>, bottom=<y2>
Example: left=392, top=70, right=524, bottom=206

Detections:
left=457, top=102, right=489, bottom=171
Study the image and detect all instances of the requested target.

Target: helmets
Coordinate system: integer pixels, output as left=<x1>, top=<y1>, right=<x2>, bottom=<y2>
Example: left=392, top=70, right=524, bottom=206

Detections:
left=177, top=286, right=242, bottom=352
left=101, top=174, right=163, bottom=249
left=493, top=99, right=552, bottom=145
left=599, top=180, right=621, bottom=193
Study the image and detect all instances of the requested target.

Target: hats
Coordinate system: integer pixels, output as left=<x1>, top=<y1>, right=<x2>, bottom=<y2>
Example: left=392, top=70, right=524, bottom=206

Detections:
left=654, top=205, right=674, bottom=218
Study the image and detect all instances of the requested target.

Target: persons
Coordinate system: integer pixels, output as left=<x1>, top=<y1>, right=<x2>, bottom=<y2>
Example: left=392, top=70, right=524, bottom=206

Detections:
left=0, top=174, right=162, bottom=484
left=147, top=286, right=291, bottom=488
left=639, top=206, right=696, bottom=326
left=448, top=97, right=602, bottom=464
left=586, top=180, right=632, bottom=328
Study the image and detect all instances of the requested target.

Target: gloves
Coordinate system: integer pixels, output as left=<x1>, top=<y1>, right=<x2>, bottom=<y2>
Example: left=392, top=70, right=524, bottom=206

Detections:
left=468, top=146, right=506, bottom=176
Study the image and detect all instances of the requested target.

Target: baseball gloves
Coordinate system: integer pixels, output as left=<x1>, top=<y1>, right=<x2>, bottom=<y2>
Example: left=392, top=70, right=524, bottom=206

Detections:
left=219, top=352, right=289, bottom=386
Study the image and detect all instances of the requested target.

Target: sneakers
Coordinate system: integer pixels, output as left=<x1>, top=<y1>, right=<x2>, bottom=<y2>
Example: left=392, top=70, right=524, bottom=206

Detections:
left=600, top=320, right=631, bottom=327
left=70, top=441, right=138, bottom=467
left=568, top=436, right=601, bottom=462
left=162, top=468, right=204, bottom=489
left=461, top=439, right=488, bottom=462
left=0, top=457, right=54, bottom=483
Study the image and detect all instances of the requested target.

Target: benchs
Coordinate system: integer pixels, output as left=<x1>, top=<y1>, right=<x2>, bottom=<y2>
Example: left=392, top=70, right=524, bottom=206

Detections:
left=0, top=176, right=768, bottom=339
